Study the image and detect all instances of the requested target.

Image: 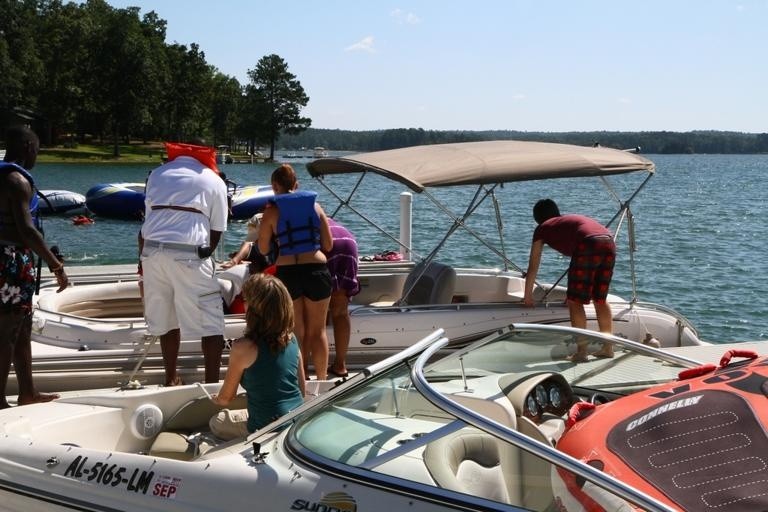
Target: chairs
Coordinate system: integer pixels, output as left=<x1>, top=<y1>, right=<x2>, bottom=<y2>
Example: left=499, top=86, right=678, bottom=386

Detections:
left=394, top=260, right=457, bottom=310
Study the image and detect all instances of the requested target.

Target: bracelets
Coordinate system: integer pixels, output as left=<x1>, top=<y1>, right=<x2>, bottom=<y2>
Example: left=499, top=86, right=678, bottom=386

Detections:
left=52, top=261, right=64, bottom=272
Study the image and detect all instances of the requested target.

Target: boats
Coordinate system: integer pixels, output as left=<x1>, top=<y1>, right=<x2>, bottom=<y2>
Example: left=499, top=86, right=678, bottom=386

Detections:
left=35, top=189, right=88, bottom=217
left=86, top=181, right=148, bottom=222
left=0, top=319, right=768, bottom=512
left=313, top=146, right=330, bottom=158
left=225, top=184, right=275, bottom=220
left=4, top=137, right=716, bottom=395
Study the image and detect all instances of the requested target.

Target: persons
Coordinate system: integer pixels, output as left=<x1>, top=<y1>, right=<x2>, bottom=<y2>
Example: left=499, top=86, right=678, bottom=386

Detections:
left=207, top=273, right=304, bottom=441
left=0, top=124, right=69, bottom=406
left=136, top=140, right=228, bottom=388
left=322, top=216, right=359, bottom=375
left=221, top=234, right=277, bottom=274
left=257, top=163, right=333, bottom=381
left=520, top=196, right=616, bottom=360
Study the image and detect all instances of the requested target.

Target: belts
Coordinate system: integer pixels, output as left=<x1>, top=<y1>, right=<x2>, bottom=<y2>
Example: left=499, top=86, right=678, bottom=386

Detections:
left=144, top=240, right=198, bottom=252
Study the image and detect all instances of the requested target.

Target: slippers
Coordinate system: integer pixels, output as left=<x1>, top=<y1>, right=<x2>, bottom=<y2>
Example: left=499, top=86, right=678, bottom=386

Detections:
left=328, top=364, right=349, bottom=377
